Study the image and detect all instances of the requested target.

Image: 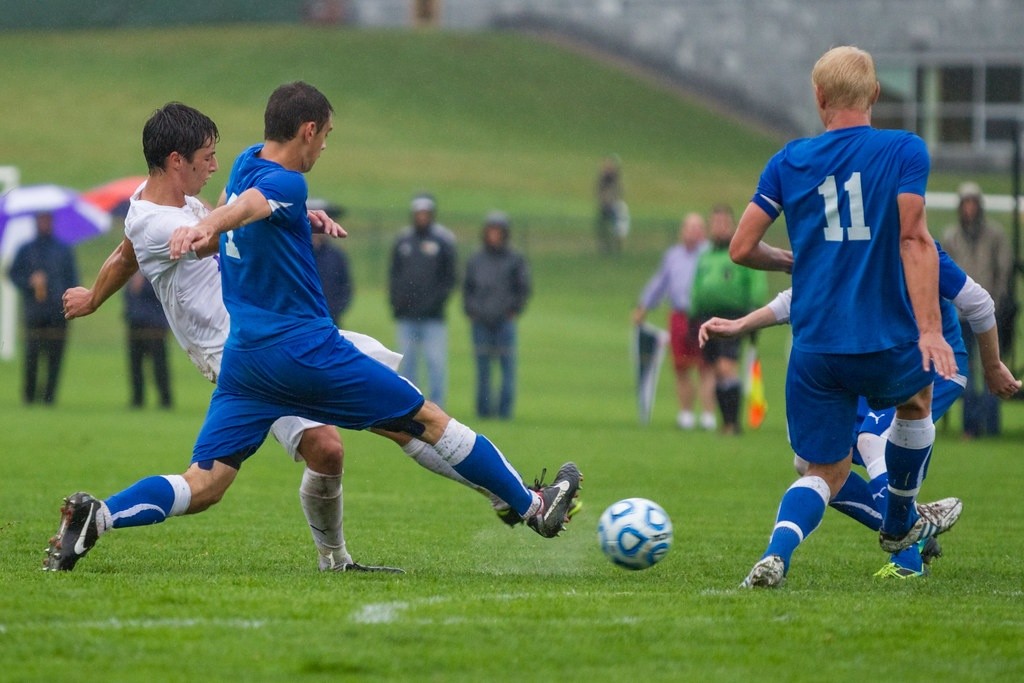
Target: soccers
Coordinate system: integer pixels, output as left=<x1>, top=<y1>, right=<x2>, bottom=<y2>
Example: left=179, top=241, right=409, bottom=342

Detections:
left=597, top=497, right=673, bottom=570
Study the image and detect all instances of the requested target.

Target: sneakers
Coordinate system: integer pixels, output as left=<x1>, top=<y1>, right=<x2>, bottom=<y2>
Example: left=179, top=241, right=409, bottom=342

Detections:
left=875, top=534, right=944, bottom=580
left=343, top=562, right=409, bottom=576
left=495, top=497, right=582, bottom=528
left=42, top=491, right=101, bottom=570
left=527, top=460, right=584, bottom=539
left=880, top=497, right=963, bottom=552
left=740, top=554, right=786, bottom=590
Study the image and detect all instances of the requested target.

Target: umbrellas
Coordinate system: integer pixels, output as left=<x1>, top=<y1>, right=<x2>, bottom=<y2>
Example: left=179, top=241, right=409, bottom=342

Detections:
left=305, top=199, right=345, bottom=218
left=77, top=176, right=213, bottom=218
left=0, top=186, right=112, bottom=255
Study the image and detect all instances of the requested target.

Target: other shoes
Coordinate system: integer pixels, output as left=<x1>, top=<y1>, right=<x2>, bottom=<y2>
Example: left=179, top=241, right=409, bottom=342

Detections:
left=699, top=411, right=719, bottom=430
left=676, top=409, right=695, bottom=431
left=724, top=421, right=739, bottom=437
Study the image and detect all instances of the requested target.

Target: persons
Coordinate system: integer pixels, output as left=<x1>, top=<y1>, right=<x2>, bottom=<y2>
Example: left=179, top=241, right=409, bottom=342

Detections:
left=44, top=80, right=584, bottom=571
left=729, top=47, right=961, bottom=588
left=312, top=234, right=353, bottom=325
left=122, top=274, right=172, bottom=408
left=631, top=207, right=767, bottom=435
left=62, top=102, right=583, bottom=574
left=595, top=156, right=624, bottom=257
left=8, top=215, right=81, bottom=404
left=387, top=194, right=459, bottom=411
left=699, top=239, right=1022, bottom=580
left=942, top=183, right=1015, bottom=438
left=462, top=214, right=531, bottom=419
